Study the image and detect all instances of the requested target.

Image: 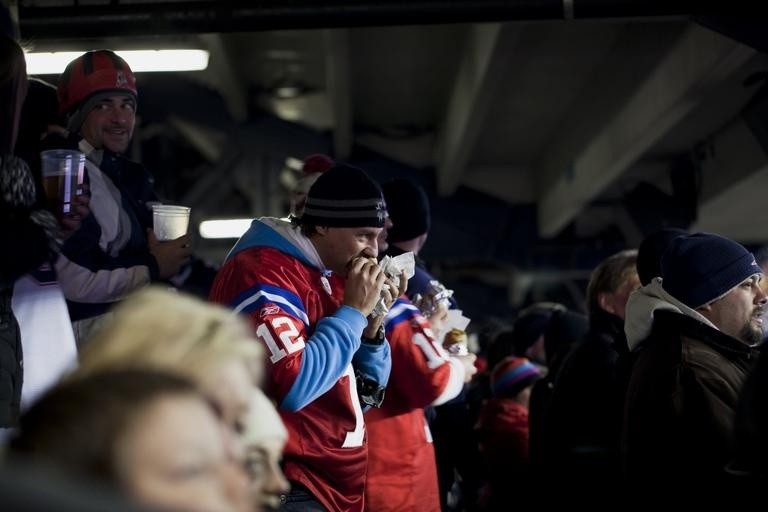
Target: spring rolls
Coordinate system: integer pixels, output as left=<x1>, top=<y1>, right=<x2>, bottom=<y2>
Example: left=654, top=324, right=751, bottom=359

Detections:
left=443, top=329, right=468, bottom=349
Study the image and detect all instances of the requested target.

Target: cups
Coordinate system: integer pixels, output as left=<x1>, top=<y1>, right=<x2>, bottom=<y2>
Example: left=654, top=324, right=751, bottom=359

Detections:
left=154, top=204, right=191, bottom=242
left=40, top=150, right=86, bottom=218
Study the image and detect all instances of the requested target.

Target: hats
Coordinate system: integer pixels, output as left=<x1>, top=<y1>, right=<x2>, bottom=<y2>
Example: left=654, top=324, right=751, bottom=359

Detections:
left=489, top=357, right=542, bottom=399
left=665, top=231, right=762, bottom=310
left=299, top=167, right=385, bottom=227
left=637, top=228, right=689, bottom=287
left=383, top=179, right=430, bottom=243
left=291, top=154, right=334, bottom=217
left=56, top=50, right=138, bottom=115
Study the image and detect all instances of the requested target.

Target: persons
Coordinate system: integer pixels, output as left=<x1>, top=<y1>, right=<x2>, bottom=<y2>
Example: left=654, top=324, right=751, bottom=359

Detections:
left=0, top=50, right=768, bottom=512
